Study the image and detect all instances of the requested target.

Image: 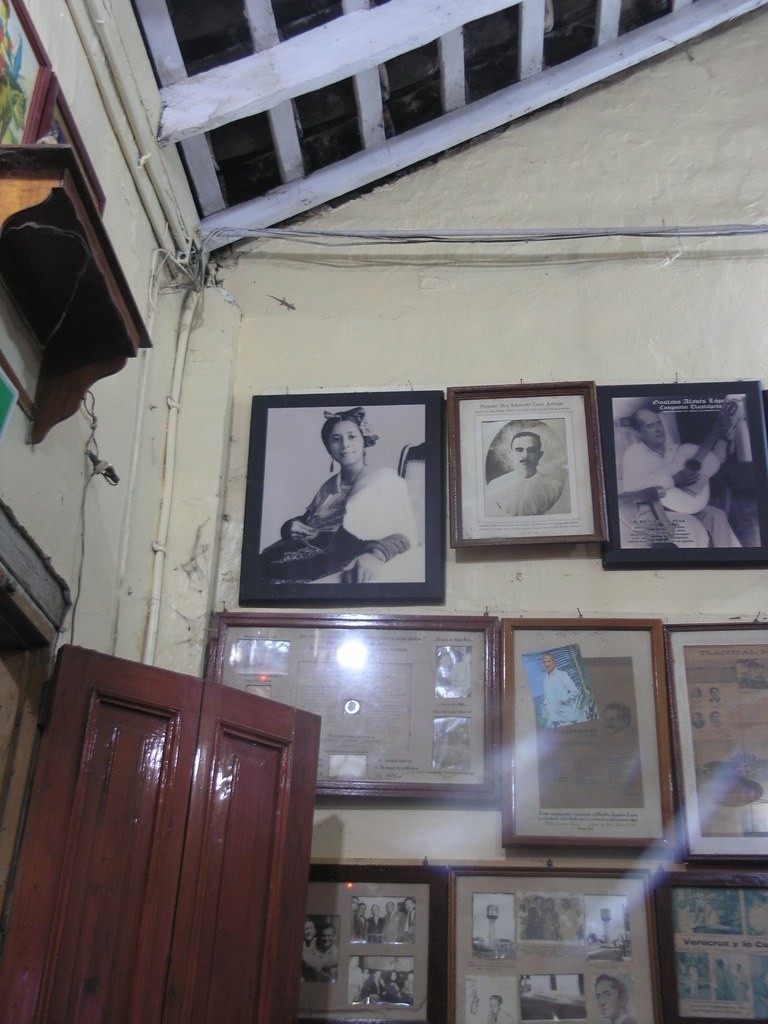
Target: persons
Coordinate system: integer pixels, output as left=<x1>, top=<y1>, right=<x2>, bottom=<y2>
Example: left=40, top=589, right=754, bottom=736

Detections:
left=475, top=893, right=632, bottom=1024
left=265, top=408, right=418, bottom=583
left=619, top=408, right=744, bottom=550
left=301, top=893, right=423, bottom=1009
left=488, top=430, right=566, bottom=515
left=540, top=653, right=585, bottom=728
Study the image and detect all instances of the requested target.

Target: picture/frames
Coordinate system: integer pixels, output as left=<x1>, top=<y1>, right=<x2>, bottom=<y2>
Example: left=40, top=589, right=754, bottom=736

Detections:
left=39, top=74, right=107, bottom=218
left=237, top=389, right=446, bottom=607
left=446, top=379, right=610, bottom=549
left=446, top=865, right=665, bottom=1024
left=210, top=612, right=502, bottom=804
left=300, top=861, right=450, bottom=1024
left=1, top=1, right=54, bottom=148
left=596, top=379, right=768, bottom=571
left=501, top=618, right=676, bottom=848
left=664, top=621, right=768, bottom=863
left=655, top=869, right=767, bottom=1024
